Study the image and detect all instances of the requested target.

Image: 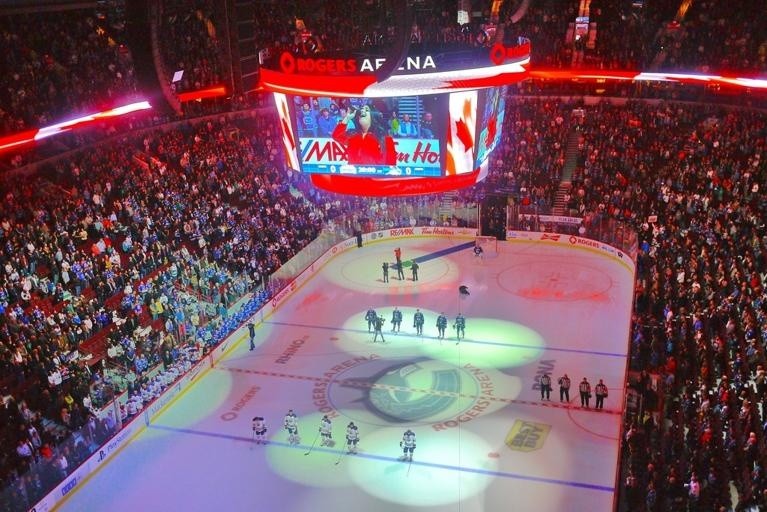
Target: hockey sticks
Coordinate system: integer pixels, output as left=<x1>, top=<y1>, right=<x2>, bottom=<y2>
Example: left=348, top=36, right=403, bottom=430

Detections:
left=334, top=438, right=347, bottom=467
left=305, top=432, right=320, bottom=455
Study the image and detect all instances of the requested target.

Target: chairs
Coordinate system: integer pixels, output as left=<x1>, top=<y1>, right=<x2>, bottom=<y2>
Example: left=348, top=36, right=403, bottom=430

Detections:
left=0, top=171, right=233, bottom=488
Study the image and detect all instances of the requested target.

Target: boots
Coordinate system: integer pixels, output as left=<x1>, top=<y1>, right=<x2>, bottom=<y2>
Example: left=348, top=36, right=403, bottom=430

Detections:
left=248, top=344, right=255, bottom=351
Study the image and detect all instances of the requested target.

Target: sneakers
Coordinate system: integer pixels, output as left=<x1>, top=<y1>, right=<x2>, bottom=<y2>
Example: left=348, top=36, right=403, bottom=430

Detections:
left=368, top=329, right=465, bottom=343
left=256, top=437, right=413, bottom=463
left=383, top=277, right=419, bottom=282
left=541, top=396, right=604, bottom=412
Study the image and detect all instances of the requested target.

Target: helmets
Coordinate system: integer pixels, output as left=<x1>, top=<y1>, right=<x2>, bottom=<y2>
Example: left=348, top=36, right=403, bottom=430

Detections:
left=588, top=394, right=592, bottom=398
left=604, top=393, right=608, bottom=398
